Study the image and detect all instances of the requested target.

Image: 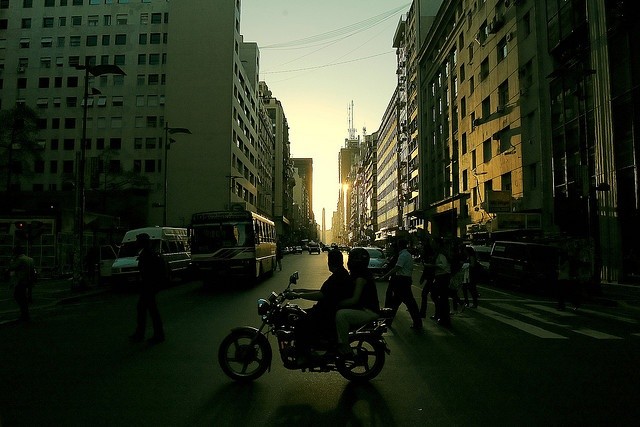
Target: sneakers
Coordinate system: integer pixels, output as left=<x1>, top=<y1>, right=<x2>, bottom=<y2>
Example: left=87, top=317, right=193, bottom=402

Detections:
left=341, top=348, right=354, bottom=358
left=450, top=310, right=459, bottom=314
left=460, top=303, right=467, bottom=310
left=150, top=337, right=165, bottom=343
left=128, top=334, right=146, bottom=341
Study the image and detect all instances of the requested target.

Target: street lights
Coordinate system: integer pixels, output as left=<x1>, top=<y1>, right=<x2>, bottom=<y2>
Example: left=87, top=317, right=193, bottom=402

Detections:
left=77, top=61, right=128, bottom=289
left=163, top=121, right=191, bottom=226
left=227, top=172, right=244, bottom=206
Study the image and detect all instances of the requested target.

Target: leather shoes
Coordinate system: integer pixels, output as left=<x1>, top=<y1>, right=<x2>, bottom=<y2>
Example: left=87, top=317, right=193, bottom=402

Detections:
left=438, top=319, right=451, bottom=324
left=470, top=305, right=478, bottom=308
left=409, top=324, right=424, bottom=329
left=419, top=314, right=426, bottom=318
left=431, top=314, right=439, bottom=318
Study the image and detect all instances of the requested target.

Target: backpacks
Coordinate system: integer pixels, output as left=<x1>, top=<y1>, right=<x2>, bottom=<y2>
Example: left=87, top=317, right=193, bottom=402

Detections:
left=144, top=252, right=173, bottom=285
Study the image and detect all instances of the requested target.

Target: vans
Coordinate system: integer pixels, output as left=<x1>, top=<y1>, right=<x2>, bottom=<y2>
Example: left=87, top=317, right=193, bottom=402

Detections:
left=98, top=226, right=192, bottom=284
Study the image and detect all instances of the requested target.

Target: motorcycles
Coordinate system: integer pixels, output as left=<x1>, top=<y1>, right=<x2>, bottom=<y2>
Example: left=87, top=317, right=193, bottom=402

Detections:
left=218, top=270, right=393, bottom=381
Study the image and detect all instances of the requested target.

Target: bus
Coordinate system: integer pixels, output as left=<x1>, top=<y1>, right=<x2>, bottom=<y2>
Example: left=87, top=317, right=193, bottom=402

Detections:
left=186, top=210, right=276, bottom=282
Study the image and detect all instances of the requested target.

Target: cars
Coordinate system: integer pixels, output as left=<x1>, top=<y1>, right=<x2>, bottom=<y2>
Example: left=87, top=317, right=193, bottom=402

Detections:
left=474, top=248, right=489, bottom=273
left=281, top=246, right=289, bottom=254
left=295, top=245, right=302, bottom=254
left=354, top=246, right=390, bottom=279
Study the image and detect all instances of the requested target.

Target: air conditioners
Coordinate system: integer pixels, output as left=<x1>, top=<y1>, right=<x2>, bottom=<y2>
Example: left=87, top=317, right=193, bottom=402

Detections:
left=506, top=34, right=512, bottom=41
left=473, top=120, right=479, bottom=125
left=497, top=105, right=504, bottom=112
left=519, top=88, right=526, bottom=95
left=491, top=13, right=503, bottom=26
left=493, top=134, right=499, bottom=140
left=487, top=21, right=494, bottom=34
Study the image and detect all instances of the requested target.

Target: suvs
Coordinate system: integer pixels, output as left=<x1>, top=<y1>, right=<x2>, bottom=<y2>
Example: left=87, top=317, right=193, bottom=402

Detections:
left=489, top=240, right=551, bottom=290
left=309, top=243, right=320, bottom=254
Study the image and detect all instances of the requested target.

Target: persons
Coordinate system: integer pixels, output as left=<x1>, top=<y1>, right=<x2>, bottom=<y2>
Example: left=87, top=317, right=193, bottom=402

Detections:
left=383, top=240, right=424, bottom=334
left=421, top=236, right=479, bottom=321
left=284, top=248, right=351, bottom=358
left=134, top=232, right=175, bottom=348
left=331, top=248, right=380, bottom=345
left=275, top=241, right=284, bottom=270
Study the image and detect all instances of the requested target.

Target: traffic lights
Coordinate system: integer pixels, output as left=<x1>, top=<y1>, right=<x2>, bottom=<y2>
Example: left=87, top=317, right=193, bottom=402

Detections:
left=15, top=220, right=25, bottom=239
left=26, top=224, right=33, bottom=232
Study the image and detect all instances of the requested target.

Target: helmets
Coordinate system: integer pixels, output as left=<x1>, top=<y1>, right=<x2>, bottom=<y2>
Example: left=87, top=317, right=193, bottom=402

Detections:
left=348, top=248, right=369, bottom=270
